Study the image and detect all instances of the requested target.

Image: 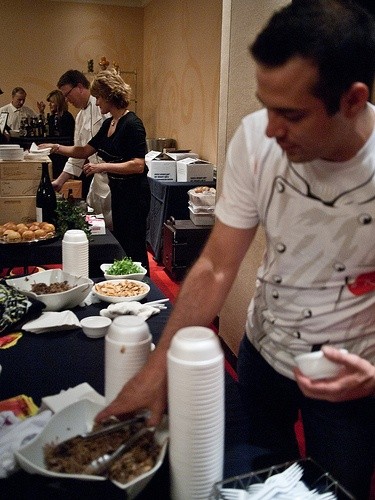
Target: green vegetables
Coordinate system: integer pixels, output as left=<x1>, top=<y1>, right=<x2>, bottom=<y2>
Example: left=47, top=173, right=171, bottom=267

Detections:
left=104, top=256, right=141, bottom=275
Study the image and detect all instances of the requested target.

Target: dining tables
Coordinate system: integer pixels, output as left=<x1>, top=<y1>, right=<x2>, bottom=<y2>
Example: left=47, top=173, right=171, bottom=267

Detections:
left=0, top=227, right=362, bottom=500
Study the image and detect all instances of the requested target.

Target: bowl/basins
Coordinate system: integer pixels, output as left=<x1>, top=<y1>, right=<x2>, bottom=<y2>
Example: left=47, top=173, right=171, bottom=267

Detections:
left=101, top=261, right=148, bottom=280
left=165, top=325, right=226, bottom=500
left=5, top=268, right=94, bottom=312
left=92, top=279, right=151, bottom=303
left=61, top=230, right=89, bottom=279
left=80, top=315, right=112, bottom=338
left=14, top=398, right=172, bottom=500
left=104, top=315, right=156, bottom=403
left=294, top=348, right=348, bottom=379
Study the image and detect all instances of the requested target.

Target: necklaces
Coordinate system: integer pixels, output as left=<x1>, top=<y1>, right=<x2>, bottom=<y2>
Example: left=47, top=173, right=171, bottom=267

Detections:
left=111, top=109, right=126, bottom=126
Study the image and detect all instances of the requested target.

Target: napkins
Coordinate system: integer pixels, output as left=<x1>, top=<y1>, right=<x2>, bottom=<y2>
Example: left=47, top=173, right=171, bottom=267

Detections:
left=81, top=285, right=98, bottom=307
left=98, top=301, right=167, bottom=323
left=22, top=310, right=82, bottom=334
left=0, top=385, right=102, bottom=481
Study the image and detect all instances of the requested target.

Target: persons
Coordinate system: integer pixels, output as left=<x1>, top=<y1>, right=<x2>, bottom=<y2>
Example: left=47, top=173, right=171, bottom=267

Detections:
left=51, top=68, right=114, bottom=234
left=0, top=88, right=11, bottom=145
left=45, top=89, right=75, bottom=178
left=92, top=0, right=375, bottom=500
left=0, top=87, right=46, bottom=134
left=36, top=70, right=150, bottom=278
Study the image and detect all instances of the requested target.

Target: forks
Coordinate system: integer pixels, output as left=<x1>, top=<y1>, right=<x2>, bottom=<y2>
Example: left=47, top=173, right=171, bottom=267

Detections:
left=220, top=461, right=337, bottom=500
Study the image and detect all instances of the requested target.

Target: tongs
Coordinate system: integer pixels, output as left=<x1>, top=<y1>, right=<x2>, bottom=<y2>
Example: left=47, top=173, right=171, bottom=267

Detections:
left=44, top=413, right=157, bottom=477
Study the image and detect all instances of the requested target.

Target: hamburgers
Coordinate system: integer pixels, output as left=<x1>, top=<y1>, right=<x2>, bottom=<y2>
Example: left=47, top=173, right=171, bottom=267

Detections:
left=0, top=222, right=55, bottom=243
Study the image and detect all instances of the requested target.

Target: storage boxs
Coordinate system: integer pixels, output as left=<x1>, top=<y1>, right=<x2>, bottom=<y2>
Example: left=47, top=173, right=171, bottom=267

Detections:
left=144, top=148, right=216, bottom=226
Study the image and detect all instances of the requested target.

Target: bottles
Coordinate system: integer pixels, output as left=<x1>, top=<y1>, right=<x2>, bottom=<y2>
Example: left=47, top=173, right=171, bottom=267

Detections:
left=19, top=112, right=51, bottom=138
left=153, top=138, right=175, bottom=160
left=35, top=162, right=60, bottom=228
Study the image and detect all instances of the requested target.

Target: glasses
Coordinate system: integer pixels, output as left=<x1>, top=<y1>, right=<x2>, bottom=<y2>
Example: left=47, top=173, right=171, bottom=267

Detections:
left=62, top=84, right=79, bottom=100
